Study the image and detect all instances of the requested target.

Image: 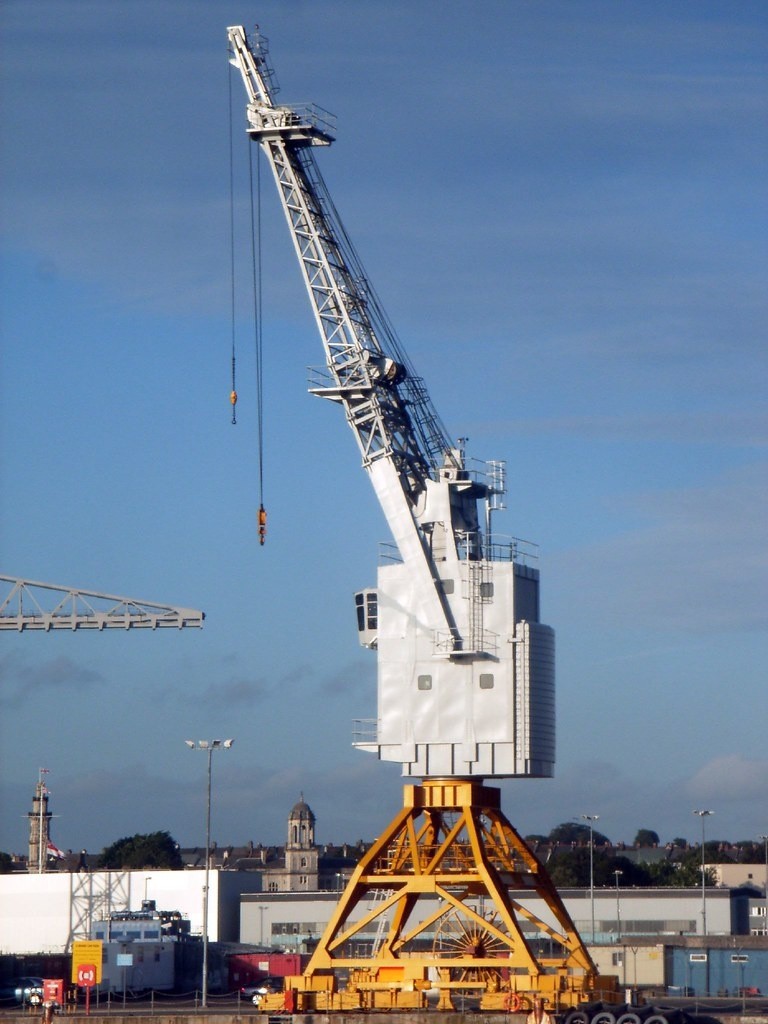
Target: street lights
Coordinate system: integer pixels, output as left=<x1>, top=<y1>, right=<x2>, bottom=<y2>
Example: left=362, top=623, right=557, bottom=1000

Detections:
left=185, top=738, right=235, bottom=1007
left=613, top=870, right=626, bottom=942
left=759, top=834, right=768, bottom=936
left=580, top=813, right=600, bottom=947
left=692, top=809, right=714, bottom=934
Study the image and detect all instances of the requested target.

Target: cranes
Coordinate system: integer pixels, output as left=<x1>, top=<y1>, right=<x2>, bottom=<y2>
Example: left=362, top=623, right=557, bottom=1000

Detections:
left=225, top=22, right=645, bottom=1018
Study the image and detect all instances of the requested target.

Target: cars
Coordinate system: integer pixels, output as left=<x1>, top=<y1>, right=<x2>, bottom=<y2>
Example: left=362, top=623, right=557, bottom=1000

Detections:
left=1, top=975, right=46, bottom=1009
left=240, top=975, right=287, bottom=1007
